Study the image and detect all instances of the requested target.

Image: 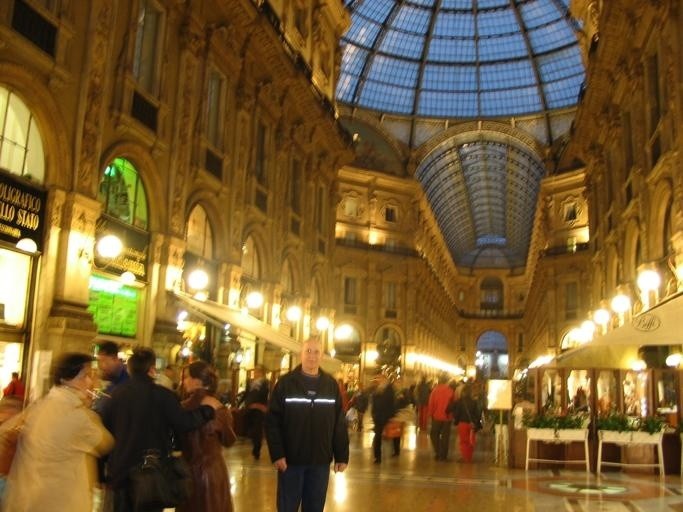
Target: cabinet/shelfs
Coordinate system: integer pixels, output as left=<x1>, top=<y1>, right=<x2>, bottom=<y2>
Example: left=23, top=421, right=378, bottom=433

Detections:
left=597, top=429, right=665, bottom=476
left=525, top=428, right=590, bottom=472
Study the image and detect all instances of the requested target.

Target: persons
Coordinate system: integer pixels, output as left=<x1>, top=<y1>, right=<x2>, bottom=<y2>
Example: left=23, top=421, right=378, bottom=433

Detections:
left=348, top=383, right=368, bottom=433
left=98, top=347, right=222, bottom=512
left=247, top=377, right=268, bottom=460
left=427, top=377, right=454, bottom=461
left=264, top=337, right=350, bottom=512
left=176, top=362, right=237, bottom=512
left=3, top=371, right=25, bottom=402
left=371, top=377, right=401, bottom=465
left=90, top=343, right=127, bottom=385
left=446, top=385, right=481, bottom=463
left=408, top=375, right=434, bottom=432
left=1, top=353, right=117, bottom=512
left=453, top=378, right=466, bottom=426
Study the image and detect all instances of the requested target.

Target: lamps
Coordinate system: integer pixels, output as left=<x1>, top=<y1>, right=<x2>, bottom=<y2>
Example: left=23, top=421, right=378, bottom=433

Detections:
left=487, top=379, right=513, bottom=472
left=173, top=270, right=209, bottom=300
left=676, top=262, right=683, bottom=291
left=570, top=320, right=595, bottom=345
left=80, top=236, right=122, bottom=270
left=231, top=288, right=263, bottom=315
left=334, top=322, right=354, bottom=341
left=629, top=360, right=647, bottom=371
left=284, top=306, right=301, bottom=323
left=666, top=352, right=682, bottom=368
left=637, top=270, right=659, bottom=310
left=314, top=314, right=329, bottom=332
left=121, top=272, right=148, bottom=285
left=611, top=294, right=631, bottom=326
left=594, top=308, right=610, bottom=335
left=16, top=238, right=42, bottom=255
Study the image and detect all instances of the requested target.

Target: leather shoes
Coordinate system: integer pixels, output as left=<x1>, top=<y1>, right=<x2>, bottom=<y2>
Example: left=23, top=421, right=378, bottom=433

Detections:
left=373, top=458, right=381, bottom=464
left=392, top=450, right=400, bottom=458
